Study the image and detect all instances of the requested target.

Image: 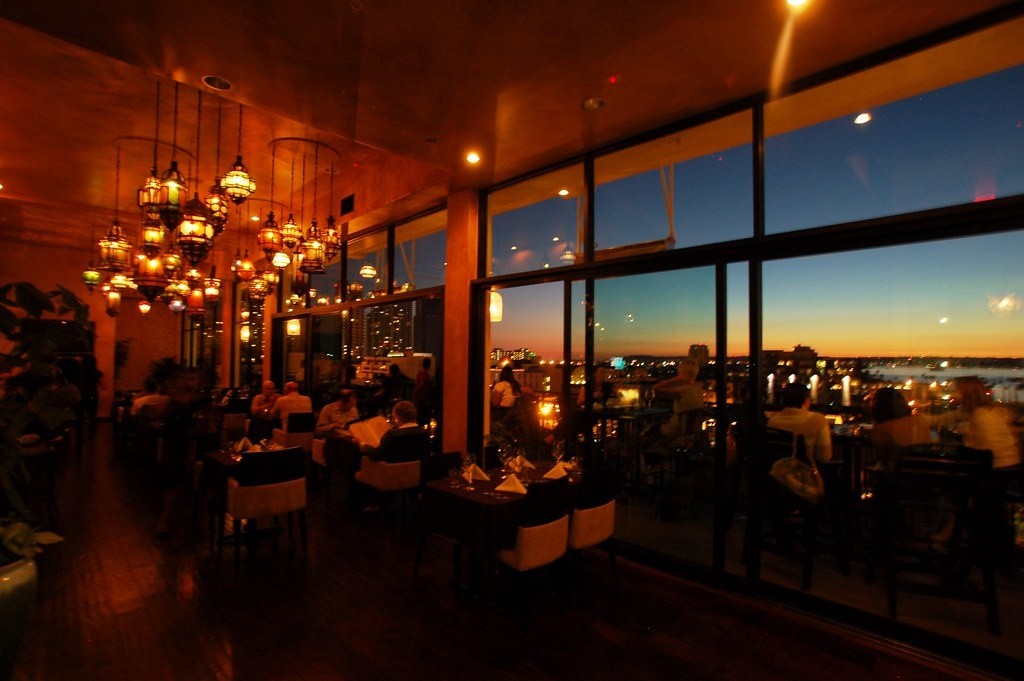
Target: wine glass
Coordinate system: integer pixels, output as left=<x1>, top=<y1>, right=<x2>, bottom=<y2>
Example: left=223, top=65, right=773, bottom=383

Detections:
left=497, top=443, right=512, bottom=471
left=551, top=439, right=565, bottom=464
left=462, top=454, right=476, bottom=493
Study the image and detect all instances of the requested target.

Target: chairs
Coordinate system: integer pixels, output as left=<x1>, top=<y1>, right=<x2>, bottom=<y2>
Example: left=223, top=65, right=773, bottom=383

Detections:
left=112, top=387, right=463, bottom=576
left=567, top=499, right=620, bottom=595
left=491, top=381, right=1023, bottom=633
left=490, top=512, right=570, bottom=633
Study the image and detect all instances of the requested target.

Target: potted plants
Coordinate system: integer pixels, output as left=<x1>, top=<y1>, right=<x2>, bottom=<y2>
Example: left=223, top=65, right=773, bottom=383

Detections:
left=0, top=280, right=109, bottom=681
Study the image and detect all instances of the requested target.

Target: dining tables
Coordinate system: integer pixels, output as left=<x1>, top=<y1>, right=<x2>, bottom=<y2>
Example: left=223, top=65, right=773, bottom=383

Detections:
left=113, top=396, right=577, bottom=592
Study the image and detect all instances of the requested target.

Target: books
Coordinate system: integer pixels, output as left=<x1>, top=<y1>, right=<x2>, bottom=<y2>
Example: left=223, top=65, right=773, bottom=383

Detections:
left=350, top=415, right=393, bottom=449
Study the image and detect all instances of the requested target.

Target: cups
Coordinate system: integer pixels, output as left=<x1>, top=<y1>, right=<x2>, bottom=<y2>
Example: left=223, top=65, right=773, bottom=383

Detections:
left=447, top=470, right=461, bottom=489
left=571, top=456, right=583, bottom=475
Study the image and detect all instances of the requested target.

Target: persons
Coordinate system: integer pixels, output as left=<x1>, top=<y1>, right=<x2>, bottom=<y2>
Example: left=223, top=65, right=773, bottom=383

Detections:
left=737, top=376, right=770, bottom=467
left=768, top=382, right=864, bottom=567
left=492, top=366, right=520, bottom=420
left=917, top=378, right=1021, bottom=557
left=870, top=385, right=932, bottom=563
left=577, top=365, right=621, bottom=408
left=111, top=355, right=434, bottom=546
left=628, top=359, right=704, bottom=486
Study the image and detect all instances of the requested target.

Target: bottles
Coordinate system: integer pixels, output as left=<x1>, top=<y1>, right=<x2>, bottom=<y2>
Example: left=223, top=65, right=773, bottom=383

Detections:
left=430, top=418, right=436, bottom=437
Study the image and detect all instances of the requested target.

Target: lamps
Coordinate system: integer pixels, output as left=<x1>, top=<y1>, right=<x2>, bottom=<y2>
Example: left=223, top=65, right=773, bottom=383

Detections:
left=79, top=74, right=344, bottom=319
